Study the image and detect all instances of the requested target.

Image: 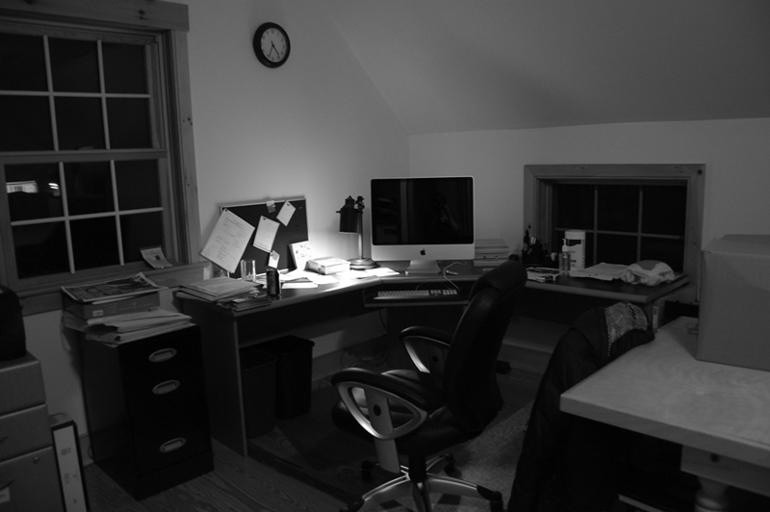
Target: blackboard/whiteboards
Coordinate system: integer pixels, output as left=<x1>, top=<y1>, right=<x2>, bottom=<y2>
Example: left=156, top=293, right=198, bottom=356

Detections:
left=219, top=197, right=308, bottom=279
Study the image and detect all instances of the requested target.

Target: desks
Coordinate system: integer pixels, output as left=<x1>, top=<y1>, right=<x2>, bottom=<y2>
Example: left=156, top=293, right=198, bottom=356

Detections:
left=558, top=309, right=770, bottom=511
left=173, top=256, right=701, bottom=457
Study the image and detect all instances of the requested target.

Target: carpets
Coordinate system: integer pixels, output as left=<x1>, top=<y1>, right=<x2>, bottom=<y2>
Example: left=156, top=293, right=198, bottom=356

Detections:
left=248, top=367, right=375, bottom=510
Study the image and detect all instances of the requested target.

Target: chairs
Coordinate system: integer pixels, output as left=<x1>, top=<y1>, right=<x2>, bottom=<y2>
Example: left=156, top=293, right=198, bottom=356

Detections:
left=330, top=257, right=527, bottom=511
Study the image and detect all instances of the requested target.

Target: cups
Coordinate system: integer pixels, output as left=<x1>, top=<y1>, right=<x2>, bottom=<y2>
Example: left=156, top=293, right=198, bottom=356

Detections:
left=557, top=251, right=569, bottom=276
left=239, top=258, right=257, bottom=283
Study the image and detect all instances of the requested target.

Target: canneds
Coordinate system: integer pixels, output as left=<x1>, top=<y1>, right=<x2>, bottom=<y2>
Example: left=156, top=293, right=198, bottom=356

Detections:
left=266, top=270, right=279, bottom=295
left=559, top=252, right=569, bottom=273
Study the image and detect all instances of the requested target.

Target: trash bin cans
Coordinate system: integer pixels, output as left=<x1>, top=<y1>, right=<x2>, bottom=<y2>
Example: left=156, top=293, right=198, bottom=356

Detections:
left=239, top=334, right=315, bottom=438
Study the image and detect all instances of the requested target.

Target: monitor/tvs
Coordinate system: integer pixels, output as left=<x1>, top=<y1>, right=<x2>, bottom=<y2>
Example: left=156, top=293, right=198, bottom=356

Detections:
left=371, top=176, right=475, bottom=273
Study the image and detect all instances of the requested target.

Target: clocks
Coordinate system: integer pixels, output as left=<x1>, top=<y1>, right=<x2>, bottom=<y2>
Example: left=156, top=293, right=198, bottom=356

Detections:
left=252, top=21, right=291, bottom=68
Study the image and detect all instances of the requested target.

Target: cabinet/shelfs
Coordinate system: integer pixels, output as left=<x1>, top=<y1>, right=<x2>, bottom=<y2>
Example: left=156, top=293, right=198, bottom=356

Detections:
left=0, top=355, right=65, bottom=511
left=65, top=326, right=216, bottom=502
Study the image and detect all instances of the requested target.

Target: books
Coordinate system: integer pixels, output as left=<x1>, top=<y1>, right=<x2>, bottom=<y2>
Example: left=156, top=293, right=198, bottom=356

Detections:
left=179, top=274, right=256, bottom=302
left=309, top=256, right=352, bottom=275
left=61, top=269, right=169, bottom=305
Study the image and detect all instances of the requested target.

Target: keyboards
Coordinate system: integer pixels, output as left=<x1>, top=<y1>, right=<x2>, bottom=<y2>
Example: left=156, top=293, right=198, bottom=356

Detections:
left=378, top=289, right=458, bottom=300
